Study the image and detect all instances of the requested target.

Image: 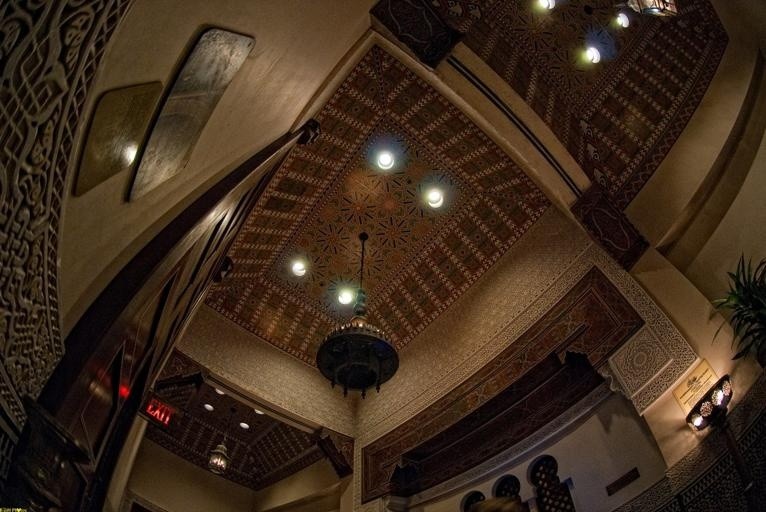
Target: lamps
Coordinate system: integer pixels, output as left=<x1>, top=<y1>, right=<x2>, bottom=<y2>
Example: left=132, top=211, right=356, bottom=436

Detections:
left=203, top=407, right=238, bottom=474
left=314, top=231, right=398, bottom=400
left=686, top=373, right=765, bottom=511
